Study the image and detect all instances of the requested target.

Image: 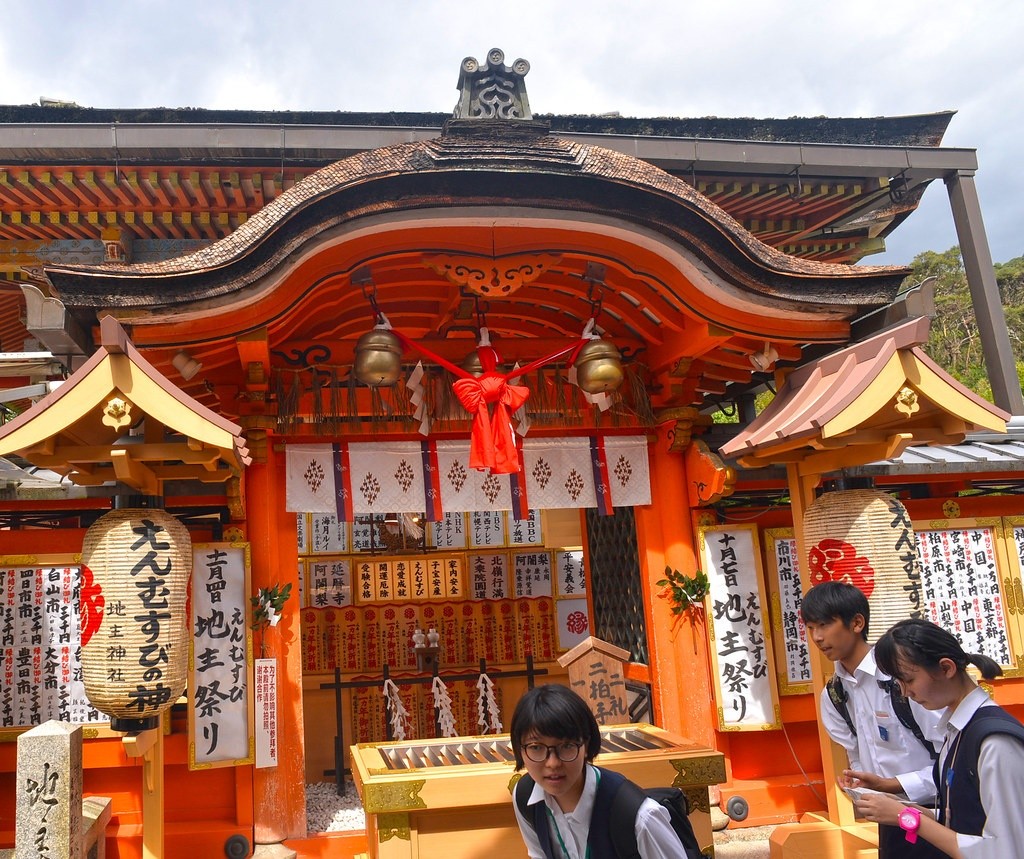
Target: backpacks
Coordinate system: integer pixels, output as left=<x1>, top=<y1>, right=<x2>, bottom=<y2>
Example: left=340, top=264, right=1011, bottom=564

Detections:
left=516, top=772, right=709, bottom=859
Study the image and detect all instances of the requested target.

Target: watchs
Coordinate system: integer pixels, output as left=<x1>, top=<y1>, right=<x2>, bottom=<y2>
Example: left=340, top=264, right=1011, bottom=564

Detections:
left=898, top=807, right=923, bottom=844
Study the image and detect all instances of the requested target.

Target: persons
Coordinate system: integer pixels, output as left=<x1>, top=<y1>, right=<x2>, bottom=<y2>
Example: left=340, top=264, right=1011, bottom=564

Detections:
left=800, top=581, right=949, bottom=859
left=837, top=619, right=1024, bottom=859
left=511, top=685, right=689, bottom=859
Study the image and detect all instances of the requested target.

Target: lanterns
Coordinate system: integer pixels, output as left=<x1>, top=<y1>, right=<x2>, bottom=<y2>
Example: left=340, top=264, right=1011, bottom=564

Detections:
left=80, top=496, right=191, bottom=730
left=803, top=477, right=924, bottom=648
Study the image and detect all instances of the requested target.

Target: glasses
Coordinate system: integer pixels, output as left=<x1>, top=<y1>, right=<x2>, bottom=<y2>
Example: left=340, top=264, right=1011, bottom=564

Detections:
left=517, top=739, right=586, bottom=764
